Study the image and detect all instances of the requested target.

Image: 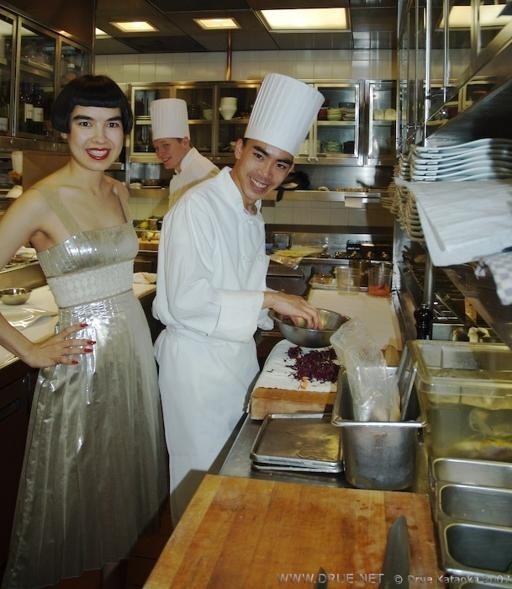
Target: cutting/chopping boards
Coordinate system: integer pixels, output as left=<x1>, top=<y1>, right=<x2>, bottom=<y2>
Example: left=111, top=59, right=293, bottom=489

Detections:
left=142, top=472, right=441, bottom=589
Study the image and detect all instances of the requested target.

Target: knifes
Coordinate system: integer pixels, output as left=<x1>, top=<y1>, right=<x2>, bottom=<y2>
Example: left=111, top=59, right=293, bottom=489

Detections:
left=379, top=513, right=412, bottom=589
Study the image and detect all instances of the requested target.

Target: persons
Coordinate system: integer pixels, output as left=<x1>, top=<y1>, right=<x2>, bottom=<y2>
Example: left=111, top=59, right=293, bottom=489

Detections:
left=0, top=75, right=169, bottom=589
left=150, top=98, right=219, bottom=208
left=151, top=71, right=323, bottom=494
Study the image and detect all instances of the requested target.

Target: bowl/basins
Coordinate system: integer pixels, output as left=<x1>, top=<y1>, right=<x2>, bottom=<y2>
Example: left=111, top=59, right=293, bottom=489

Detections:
left=267, top=308, right=348, bottom=348
left=220, top=97, right=236, bottom=119
left=0, top=288, right=31, bottom=306
left=431, top=455, right=511, bottom=492
left=440, top=522, right=511, bottom=581
left=330, top=364, right=427, bottom=488
left=435, top=483, right=512, bottom=526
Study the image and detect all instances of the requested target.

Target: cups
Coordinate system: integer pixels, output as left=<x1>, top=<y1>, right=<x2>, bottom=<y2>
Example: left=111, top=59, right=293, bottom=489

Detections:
left=370, top=268, right=391, bottom=296
left=336, top=266, right=361, bottom=296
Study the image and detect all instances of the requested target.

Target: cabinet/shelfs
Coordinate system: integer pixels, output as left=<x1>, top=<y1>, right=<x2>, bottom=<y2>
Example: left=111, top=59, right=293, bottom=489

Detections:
left=0, top=4, right=97, bottom=156
left=422, top=78, right=505, bottom=136
left=366, top=80, right=406, bottom=161
left=126, top=80, right=363, bottom=168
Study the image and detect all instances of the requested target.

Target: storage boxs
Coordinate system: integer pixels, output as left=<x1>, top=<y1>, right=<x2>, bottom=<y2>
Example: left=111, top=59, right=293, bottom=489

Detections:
left=410, top=339, right=512, bottom=462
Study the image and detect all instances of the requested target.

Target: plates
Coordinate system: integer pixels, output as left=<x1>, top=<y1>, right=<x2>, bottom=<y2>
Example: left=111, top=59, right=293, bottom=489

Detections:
left=391, top=154, right=409, bottom=228
left=327, top=108, right=342, bottom=120
left=249, top=412, right=344, bottom=473
left=408, top=134, right=512, bottom=239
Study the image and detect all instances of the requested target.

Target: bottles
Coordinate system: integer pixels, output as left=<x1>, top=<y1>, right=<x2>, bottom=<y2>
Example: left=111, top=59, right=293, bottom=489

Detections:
left=32, top=83, right=44, bottom=135
left=20, top=80, right=34, bottom=132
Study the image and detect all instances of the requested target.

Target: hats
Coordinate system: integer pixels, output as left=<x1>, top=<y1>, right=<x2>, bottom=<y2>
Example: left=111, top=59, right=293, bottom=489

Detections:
left=149, top=97, right=190, bottom=143
left=243, top=72, right=326, bottom=158
